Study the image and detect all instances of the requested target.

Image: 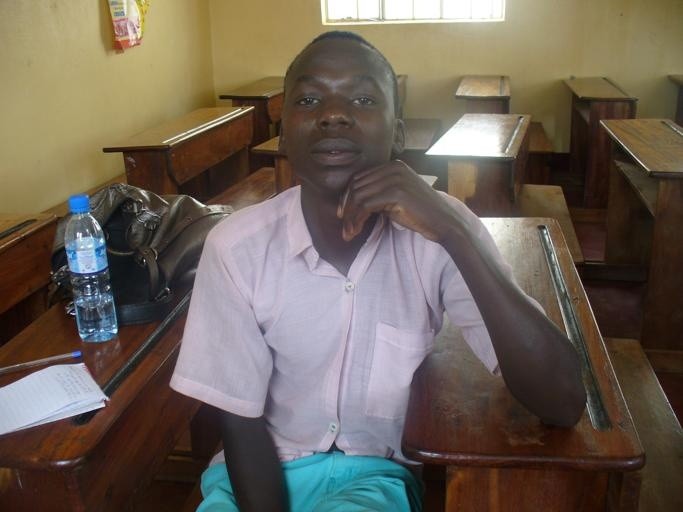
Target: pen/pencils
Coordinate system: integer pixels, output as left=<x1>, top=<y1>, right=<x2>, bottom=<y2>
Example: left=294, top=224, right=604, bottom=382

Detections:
left=1, top=350, right=82, bottom=374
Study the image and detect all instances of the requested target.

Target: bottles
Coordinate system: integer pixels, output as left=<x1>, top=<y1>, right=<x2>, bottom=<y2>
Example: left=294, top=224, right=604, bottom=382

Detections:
left=63, top=193, right=121, bottom=344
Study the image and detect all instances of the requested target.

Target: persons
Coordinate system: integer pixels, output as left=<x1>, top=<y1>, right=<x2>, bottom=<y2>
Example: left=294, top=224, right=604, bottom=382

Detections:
left=167, top=31, right=588, bottom=512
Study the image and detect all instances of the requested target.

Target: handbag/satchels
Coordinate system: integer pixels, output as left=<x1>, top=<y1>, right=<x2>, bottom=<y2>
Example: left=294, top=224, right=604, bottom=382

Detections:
left=49, top=182, right=236, bottom=326
